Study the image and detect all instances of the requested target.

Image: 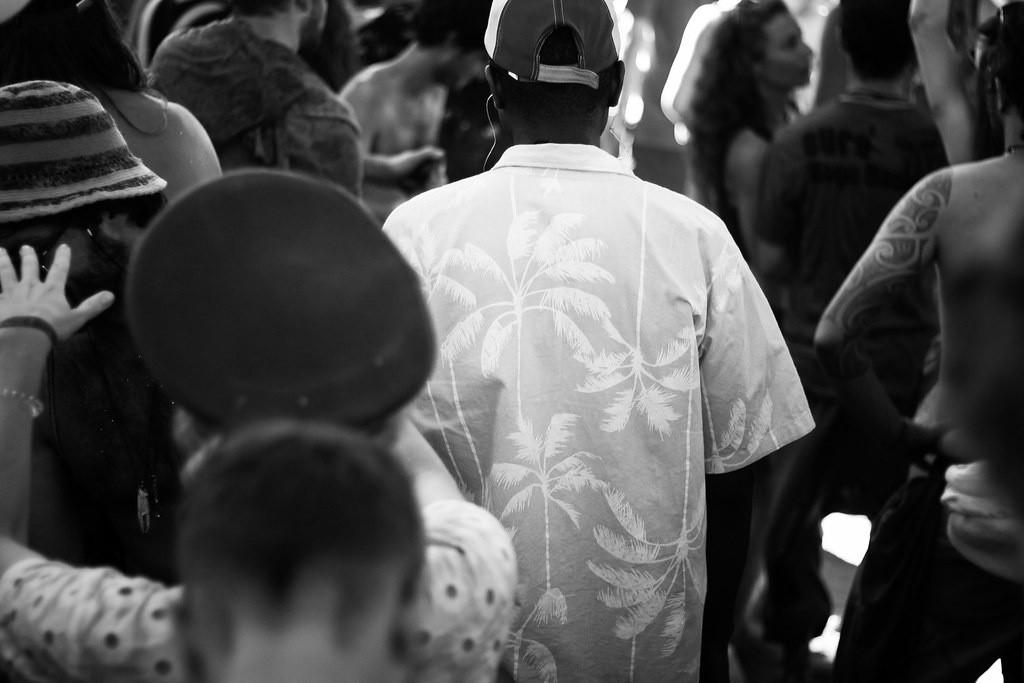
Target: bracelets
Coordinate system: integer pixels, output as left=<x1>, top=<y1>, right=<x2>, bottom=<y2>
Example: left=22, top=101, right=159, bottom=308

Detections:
left=0, top=316, right=56, bottom=344
left=0, top=389, right=43, bottom=417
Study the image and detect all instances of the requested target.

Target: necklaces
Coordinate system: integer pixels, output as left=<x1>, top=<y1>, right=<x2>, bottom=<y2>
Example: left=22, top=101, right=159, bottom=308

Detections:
left=1006, top=144, right=1024, bottom=152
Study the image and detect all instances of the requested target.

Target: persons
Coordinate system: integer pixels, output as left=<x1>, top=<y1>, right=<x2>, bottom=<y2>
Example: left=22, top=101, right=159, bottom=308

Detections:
left=0, top=171, right=517, bottom=683
left=177, top=424, right=426, bottom=683
left=383, top=0, right=815, bottom=683
left=616, top=0, right=1004, bottom=683
left=0, top=0, right=490, bottom=587
left=814, top=0, right=1024, bottom=683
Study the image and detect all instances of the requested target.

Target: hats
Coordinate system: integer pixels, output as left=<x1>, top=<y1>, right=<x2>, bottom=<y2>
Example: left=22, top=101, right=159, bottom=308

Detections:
left=124, top=168, right=437, bottom=428
left=483, top=0, right=619, bottom=91
left=0, top=79, right=170, bottom=224
